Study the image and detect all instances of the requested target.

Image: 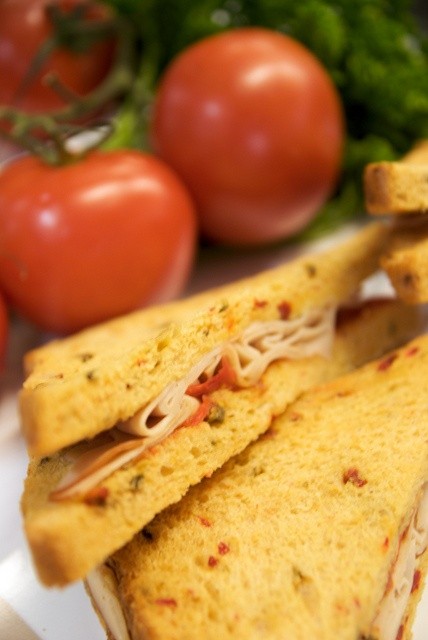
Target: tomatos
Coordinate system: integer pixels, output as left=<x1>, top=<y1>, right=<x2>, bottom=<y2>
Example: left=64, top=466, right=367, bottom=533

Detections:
left=0, top=0, right=122, bottom=141
left=1, top=149, right=199, bottom=336
left=152, top=28, right=347, bottom=248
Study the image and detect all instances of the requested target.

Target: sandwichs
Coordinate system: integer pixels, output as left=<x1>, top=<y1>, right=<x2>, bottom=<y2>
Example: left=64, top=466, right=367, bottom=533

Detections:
left=364, top=137, right=428, bottom=306
left=18, top=220, right=428, bottom=640
